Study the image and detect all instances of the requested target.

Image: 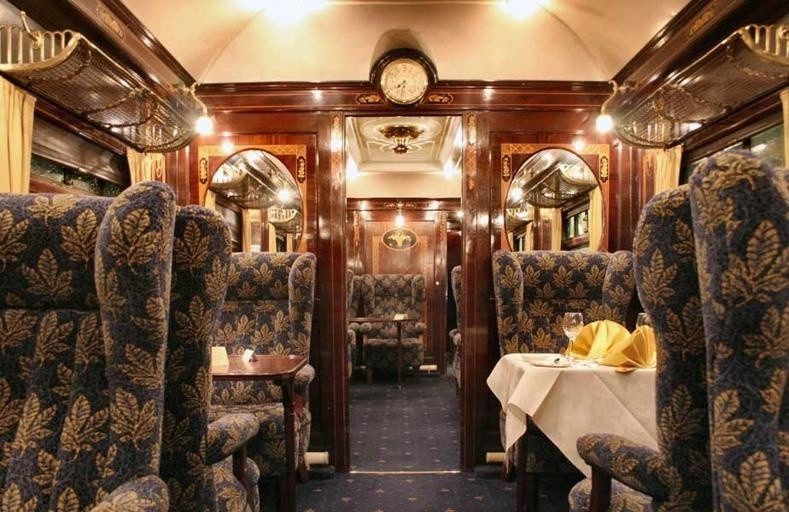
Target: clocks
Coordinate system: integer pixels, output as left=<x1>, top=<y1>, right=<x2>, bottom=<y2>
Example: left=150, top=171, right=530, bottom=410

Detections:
left=367, top=47, right=439, bottom=111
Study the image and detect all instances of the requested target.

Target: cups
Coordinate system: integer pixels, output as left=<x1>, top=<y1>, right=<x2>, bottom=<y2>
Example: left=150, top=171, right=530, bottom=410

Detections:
left=636, top=313, right=653, bottom=328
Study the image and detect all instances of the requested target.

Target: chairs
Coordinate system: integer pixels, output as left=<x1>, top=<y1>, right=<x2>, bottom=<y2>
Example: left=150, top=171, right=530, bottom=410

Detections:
left=490, top=247, right=635, bottom=507
left=204, top=252, right=317, bottom=505
left=567, top=182, right=712, bottom=508
left=360, top=273, right=428, bottom=383
left=0, top=180, right=260, bottom=506
left=346, top=270, right=358, bottom=377
left=448, top=265, right=462, bottom=395
left=688, top=153, right=788, bottom=509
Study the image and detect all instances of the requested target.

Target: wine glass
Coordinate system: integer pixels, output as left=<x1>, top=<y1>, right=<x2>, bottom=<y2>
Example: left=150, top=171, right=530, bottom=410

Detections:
left=562, top=312, right=585, bottom=366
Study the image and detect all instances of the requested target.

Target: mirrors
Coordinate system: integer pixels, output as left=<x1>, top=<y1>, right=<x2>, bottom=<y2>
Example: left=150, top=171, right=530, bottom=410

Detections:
left=197, top=142, right=308, bottom=253
left=500, top=140, right=613, bottom=255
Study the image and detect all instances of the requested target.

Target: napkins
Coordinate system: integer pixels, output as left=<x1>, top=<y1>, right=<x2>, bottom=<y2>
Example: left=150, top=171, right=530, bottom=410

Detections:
left=564, top=319, right=632, bottom=365
left=598, top=325, right=657, bottom=369
